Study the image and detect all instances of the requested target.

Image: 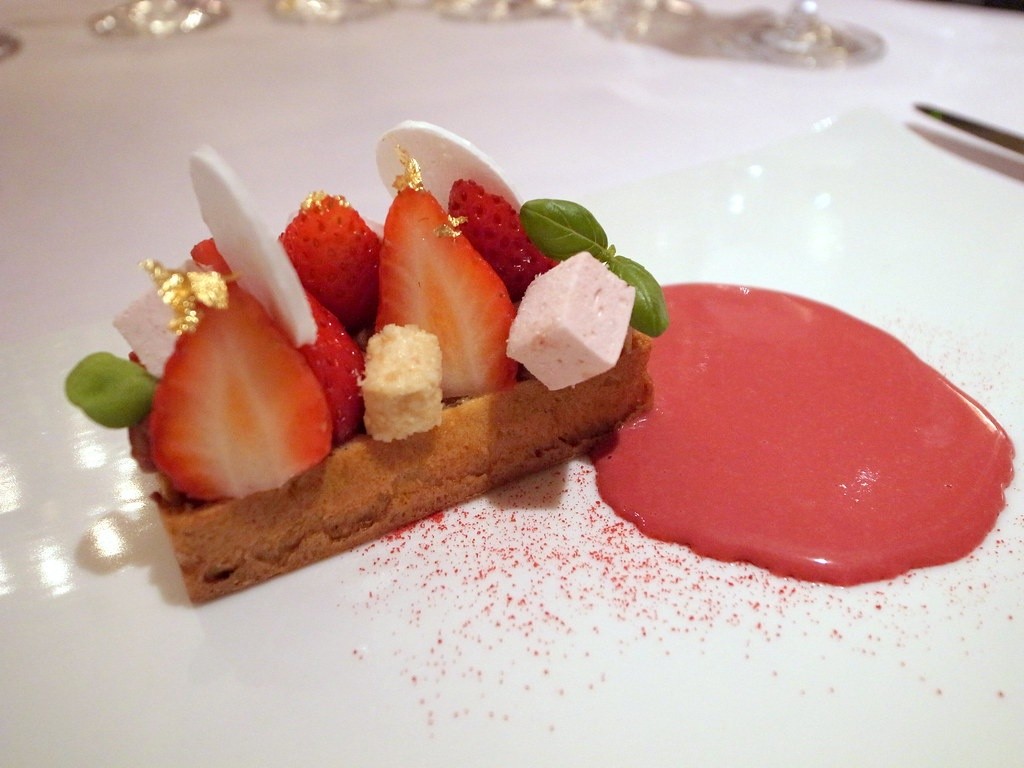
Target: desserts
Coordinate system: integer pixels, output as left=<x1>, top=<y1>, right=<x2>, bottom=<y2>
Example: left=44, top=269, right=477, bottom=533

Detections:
left=60, top=119, right=655, bottom=612
